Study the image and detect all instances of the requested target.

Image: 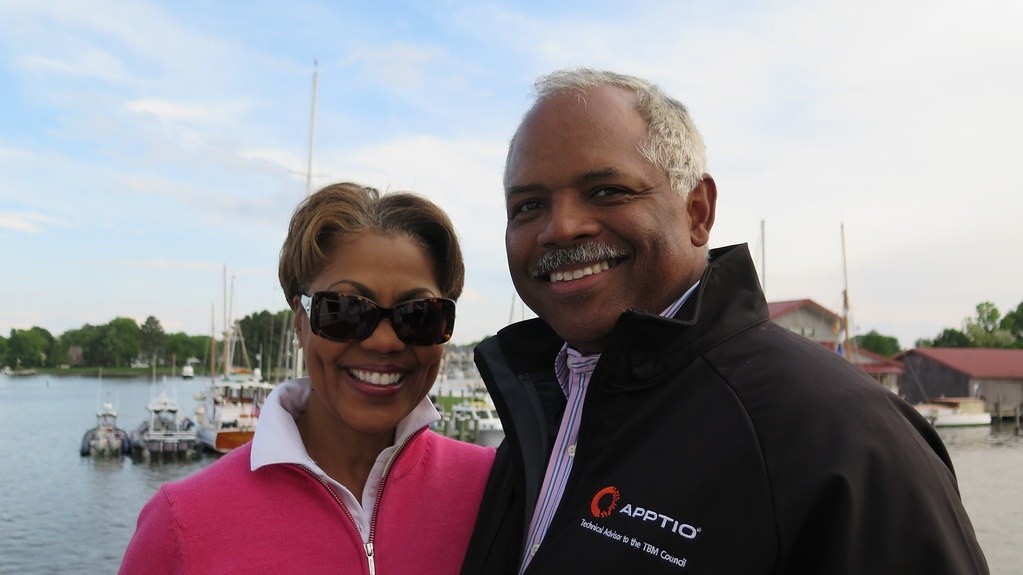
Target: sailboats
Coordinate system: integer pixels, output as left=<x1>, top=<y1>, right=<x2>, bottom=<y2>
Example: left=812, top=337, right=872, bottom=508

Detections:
left=191, top=56, right=505, bottom=460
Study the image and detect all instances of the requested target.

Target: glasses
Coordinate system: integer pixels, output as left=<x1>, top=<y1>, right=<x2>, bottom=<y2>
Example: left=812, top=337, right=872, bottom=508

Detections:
left=300, top=289, right=456, bottom=349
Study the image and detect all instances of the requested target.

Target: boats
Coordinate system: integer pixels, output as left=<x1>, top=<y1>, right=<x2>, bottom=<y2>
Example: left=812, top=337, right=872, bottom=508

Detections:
left=129, top=350, right=202, bottom=466
left=78, top=368, right=130, bottom=462
left=908, top=396, right=993, bottom=429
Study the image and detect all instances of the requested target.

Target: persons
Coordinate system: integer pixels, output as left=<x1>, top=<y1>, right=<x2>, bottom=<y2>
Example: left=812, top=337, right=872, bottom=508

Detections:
left=429, top=396, right=443, bottom=431
left=457, top=69, right=992, bottom=575
left=118, top=181, right=495, bottom=575
